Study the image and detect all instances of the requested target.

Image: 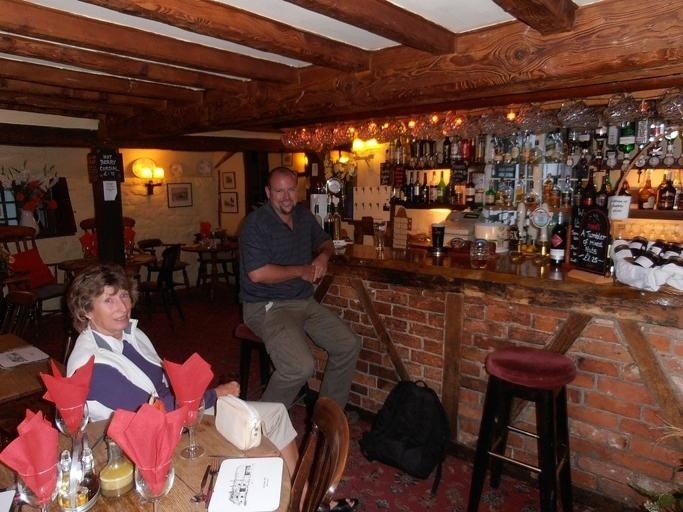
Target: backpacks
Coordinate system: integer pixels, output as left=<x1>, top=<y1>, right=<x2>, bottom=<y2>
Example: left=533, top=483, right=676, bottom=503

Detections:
left=359, top=380, right=452, bottom=495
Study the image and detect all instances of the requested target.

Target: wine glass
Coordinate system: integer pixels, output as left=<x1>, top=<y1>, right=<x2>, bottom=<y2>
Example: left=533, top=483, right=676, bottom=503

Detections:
left=280, top=86, right=682, bottom=150
left=134, top=458, right=174, bottom=512
left=175, top=396, right=207, bottom=460
left=17, top=462, right=63, bottom=512
left=54, top=402, right=90, bottom=440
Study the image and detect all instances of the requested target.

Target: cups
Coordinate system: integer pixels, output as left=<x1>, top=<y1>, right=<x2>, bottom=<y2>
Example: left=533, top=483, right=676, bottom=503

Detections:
left=432, top=252, right=445, bottom=267
left=373, top=217, right=387, bottom=251
left=432, top=223, right=446, bottom=251
left=469, top=239, right=490, bottom=261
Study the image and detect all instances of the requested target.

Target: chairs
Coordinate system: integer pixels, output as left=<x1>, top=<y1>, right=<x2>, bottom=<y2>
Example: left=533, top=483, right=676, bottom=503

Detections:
left=287, top=396, right=349, bottom=511
left=0, top=218, right=239, bottom=364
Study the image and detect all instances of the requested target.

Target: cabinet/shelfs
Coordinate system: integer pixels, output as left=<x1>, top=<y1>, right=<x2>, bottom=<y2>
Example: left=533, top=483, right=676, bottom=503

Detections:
left=390, top=134, right=683, bottom=253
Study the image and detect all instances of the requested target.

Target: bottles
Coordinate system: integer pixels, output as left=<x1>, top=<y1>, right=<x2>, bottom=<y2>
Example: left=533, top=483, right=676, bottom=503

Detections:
left=544, top=168, right=683, bottom=212
left=57, top=440, right=97, bottom=509
left=98, top=437, right=135, bottom=498
left=448, top=177, right=537, bottom=205
left=549, top=212, right=567, bottom=266
left=314, top=204, right=341, bottom=240
left=389, top=118, right=679, bottom=163
left=549, top=266, right=564, bottom=281
left=408, top=171, right=446, bottom=203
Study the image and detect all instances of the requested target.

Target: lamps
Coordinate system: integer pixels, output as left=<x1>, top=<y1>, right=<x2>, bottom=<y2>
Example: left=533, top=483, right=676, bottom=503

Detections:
left=140, top=167, right=164, bottom=195
left=352, top=138, right=381, bottom=160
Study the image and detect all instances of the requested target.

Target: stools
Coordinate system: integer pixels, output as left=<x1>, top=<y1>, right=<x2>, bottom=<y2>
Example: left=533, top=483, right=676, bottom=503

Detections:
left=466, top=347, right=576, bottom=511
left=236, top=324, right=311, bottom=414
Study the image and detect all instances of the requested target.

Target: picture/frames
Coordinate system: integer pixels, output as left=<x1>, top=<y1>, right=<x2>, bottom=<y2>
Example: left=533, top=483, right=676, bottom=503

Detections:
left=222, top=171, right=236, bottom=189
left=167, top=183, right=192, bottom=207
left=220, top=192, right=238, bottom=213
left=281, top=153, right=293, bottom=166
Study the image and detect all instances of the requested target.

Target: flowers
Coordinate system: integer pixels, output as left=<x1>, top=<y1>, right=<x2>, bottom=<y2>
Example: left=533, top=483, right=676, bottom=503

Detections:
left=0, top=159, right=59, bottom=212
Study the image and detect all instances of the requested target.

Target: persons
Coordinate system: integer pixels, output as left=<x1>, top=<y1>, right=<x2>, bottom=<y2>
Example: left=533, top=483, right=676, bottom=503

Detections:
left=62, top=262, right=362, bottom=512
left=235, top=165, right=362, bottom=412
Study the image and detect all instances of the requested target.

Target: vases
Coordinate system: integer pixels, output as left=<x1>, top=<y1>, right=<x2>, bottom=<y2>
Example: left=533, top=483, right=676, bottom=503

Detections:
left=18, top=207, right=41, bottom=239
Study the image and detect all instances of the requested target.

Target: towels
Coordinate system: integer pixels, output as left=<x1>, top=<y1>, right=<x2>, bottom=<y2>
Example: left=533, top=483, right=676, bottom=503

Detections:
left=39, top=355, right=94, bottom=431
left=1, top=408, right=59, bottom=504
left=163, top=352, right=214, bottom=423
left=107, top=398, right=189, bottom=497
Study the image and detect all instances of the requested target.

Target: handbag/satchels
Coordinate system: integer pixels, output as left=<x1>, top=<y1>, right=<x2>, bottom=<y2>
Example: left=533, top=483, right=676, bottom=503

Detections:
left=213, top=393, right=262, bottom=450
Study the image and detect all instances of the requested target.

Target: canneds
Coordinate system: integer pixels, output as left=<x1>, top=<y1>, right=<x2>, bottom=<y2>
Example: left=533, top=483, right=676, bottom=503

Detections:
left=614, top=236, right=683, bottom=270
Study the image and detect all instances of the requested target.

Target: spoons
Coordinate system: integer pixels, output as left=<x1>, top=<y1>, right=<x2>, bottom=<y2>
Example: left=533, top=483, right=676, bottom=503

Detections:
left=190, top=464, right=210, bottom=504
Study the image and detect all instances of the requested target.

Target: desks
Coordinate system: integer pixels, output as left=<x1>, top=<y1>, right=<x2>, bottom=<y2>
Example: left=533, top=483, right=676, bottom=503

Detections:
left=1, top=332, right=71, bottom=402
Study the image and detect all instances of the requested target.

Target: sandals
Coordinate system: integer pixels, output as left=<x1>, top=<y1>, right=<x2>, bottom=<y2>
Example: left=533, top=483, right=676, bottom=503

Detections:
left=330, top=497, right=360, bottom=512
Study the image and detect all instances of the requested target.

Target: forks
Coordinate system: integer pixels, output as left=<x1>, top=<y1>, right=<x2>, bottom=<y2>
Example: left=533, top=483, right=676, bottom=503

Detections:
left=205, top=458, right=220, bottom=508
